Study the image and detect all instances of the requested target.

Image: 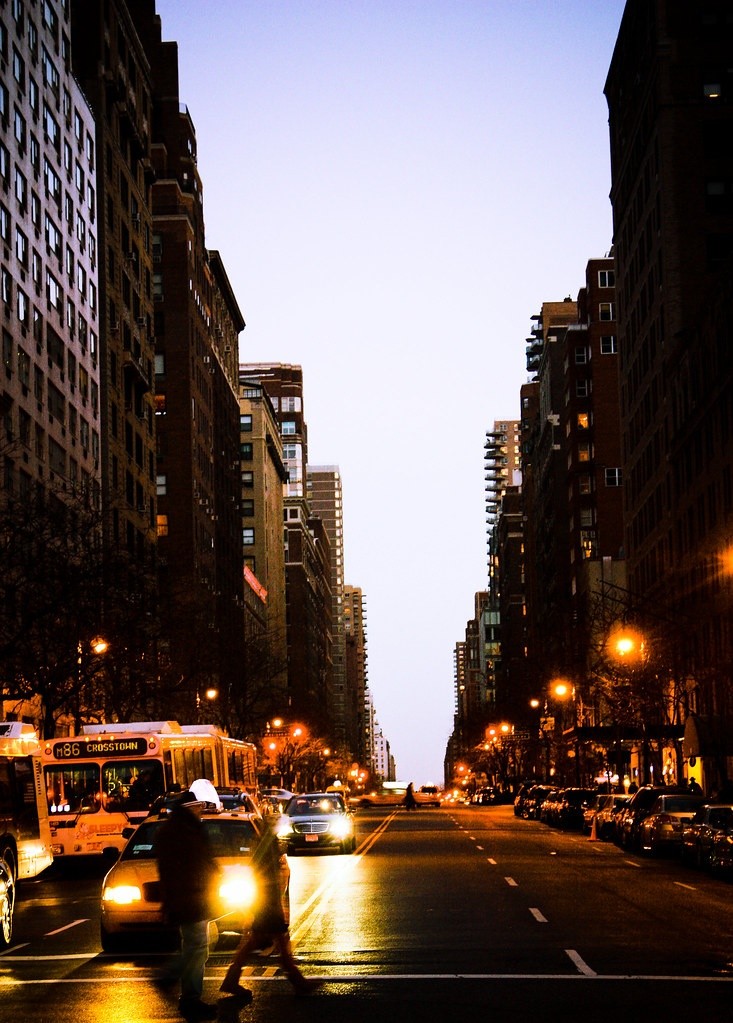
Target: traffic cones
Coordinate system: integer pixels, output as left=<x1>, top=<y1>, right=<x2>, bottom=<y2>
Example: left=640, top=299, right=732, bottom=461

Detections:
left=587, top=816, right=600, bottom=840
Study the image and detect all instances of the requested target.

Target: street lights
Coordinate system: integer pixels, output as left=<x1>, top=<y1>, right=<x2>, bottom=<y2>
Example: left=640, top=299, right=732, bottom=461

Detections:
left=490, top=683, right=581, bottom=787
left=74, top=641, right=109, bottom=736
left=190, top=689, right=367, bottom=794
left=616, top=638, right=651, bottom=787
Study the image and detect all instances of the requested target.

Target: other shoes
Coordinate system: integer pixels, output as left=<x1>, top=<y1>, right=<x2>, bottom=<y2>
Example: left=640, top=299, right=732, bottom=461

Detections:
left=157, top=977, right=178, bottom=1000
left=293, top=978, right=325, bottom=992
left=178, top=999, right=219, bottom=1013
left=220, top=982, right=252, bottom=996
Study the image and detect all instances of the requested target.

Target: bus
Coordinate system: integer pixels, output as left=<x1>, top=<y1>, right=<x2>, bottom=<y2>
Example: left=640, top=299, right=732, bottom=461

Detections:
left=0, top=721, right=55, bottom=886
left=41, top=720, right=259, bottom=857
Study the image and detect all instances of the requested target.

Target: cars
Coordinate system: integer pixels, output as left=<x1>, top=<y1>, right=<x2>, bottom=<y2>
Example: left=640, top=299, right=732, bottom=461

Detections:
left=102, top=788, right=358, bottom=952
left=417, top=785, right=441, bottom=807
left=354, top=788, right=428, bottom=809
left=513, top=782, right=733, bottom=869
left=472, top=786, right=502, bottom=804
left=0, top=856, right=16, bottom=945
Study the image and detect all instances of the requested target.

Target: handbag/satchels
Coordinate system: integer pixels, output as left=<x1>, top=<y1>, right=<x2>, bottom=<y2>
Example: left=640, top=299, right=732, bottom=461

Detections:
left=144, top=881, right=161, bottom=902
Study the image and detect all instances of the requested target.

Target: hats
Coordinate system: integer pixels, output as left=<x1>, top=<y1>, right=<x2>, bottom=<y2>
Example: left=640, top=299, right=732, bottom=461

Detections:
left=179, top=792, right=202, bottom=806
left=262, top=803, right=281, bottom=816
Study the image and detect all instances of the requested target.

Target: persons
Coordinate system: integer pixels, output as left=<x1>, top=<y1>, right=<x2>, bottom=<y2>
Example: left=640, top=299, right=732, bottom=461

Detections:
left=406, top=782, right=416, bottom=811
left=154, top=792, right=217, bottom=1016
left=219, top=812, right=313, bottom=994
left=49, top=770, right=283, bottom=813
left=519, top=774, right=733, bottom=804
